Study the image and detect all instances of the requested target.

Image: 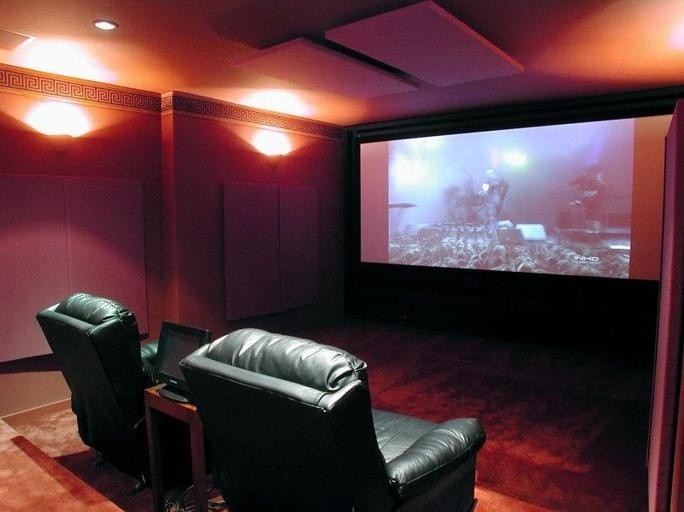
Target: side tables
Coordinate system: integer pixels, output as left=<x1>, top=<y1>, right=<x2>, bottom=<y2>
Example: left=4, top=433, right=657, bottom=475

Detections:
left=139, top=384, right=209, bottom=512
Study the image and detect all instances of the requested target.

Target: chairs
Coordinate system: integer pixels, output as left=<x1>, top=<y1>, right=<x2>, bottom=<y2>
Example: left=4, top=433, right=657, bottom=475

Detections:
left=34, top=290, right=158, bottom=490
left=178, top=326, right=485, bottom=510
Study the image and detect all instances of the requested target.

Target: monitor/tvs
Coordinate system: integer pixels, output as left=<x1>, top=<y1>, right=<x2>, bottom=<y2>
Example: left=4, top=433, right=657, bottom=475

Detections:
left=153, top=320, right=218, bottom=402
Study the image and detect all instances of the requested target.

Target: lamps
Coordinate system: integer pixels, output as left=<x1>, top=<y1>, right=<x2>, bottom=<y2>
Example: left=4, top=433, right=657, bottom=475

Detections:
left=234, top=1, right=524, bottom=101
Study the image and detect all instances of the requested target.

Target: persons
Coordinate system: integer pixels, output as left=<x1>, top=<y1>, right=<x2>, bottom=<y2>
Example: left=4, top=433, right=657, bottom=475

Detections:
left=470, top=167, right=510, bottom=233
